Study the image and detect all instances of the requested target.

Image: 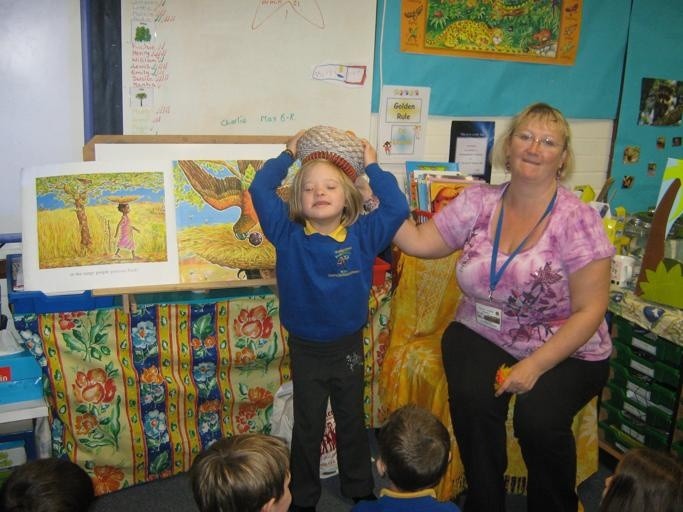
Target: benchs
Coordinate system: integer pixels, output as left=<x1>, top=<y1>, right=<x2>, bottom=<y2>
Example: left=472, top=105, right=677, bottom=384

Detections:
left=381, top=247, right=598, bottom=511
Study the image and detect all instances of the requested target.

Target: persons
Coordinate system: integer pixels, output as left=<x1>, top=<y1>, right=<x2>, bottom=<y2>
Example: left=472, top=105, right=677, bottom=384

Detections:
left=390, top=102, right=616, bottom=511
left=186, top=433, right=294, bottom=512
left=1, top=456, right=96, bottom=510
left=247, top=126, right=412, bottom=512
left=595, top=445, right=683, bottom=512
left=347, top=403, right=463, bottom=512
left=640, top=79, right=682, bottom=127
left=112, top=202, right=145, bottom=261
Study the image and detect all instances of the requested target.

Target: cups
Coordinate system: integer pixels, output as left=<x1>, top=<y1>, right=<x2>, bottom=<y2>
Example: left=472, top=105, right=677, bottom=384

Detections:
left=611, top=254, right=634, bottom=287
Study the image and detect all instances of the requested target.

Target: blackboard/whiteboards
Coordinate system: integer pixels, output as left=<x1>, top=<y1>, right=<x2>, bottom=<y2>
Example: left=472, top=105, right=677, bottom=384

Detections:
left=120, top=0, right=377, bottom=141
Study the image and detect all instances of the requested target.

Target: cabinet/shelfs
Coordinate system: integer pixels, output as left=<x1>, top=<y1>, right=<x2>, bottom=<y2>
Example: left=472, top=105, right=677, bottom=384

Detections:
left=598, top=281, right=682, bottom=463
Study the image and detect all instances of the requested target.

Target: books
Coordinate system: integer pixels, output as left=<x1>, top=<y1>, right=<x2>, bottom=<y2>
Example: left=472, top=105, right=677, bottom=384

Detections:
left=404, top=157, right=487, bottom=226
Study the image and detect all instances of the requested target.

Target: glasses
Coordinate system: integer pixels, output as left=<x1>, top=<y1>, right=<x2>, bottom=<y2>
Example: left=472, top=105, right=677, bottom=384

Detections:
left=513, top=130, right=562, bottom=151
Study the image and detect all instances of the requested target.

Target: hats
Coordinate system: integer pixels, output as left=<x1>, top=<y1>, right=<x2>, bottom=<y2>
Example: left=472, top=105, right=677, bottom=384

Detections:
left=296, top=125, right=366, bottom=183
